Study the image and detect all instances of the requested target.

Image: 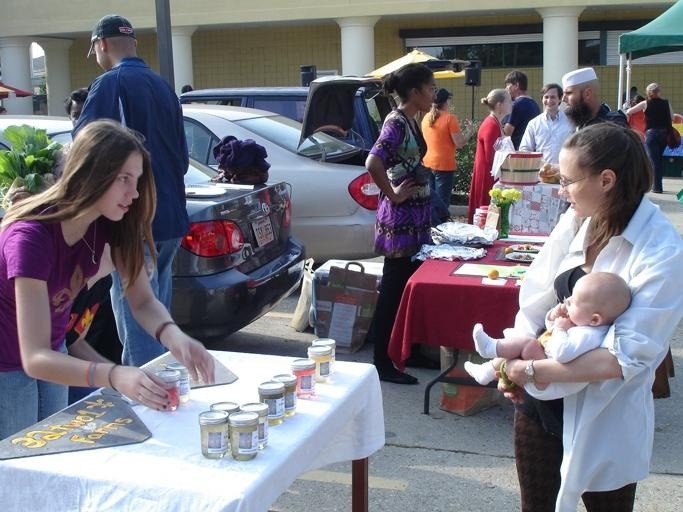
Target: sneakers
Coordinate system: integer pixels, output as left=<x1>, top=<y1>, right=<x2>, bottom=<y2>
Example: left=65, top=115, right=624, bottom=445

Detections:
left=376, top=364, right=417, bottom=384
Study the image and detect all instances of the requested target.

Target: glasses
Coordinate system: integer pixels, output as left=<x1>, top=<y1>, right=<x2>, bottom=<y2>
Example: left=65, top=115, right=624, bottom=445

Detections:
left=560, top=178, right=585, bottom=188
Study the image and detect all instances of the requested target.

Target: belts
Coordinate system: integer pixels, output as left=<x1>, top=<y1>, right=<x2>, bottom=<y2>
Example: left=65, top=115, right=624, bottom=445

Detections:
left=647, top=129, right=666, bottom=132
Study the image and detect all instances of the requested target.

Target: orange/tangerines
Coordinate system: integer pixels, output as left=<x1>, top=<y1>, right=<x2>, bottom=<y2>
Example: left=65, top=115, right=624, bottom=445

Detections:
left=488, top=269, right=499, bottom=280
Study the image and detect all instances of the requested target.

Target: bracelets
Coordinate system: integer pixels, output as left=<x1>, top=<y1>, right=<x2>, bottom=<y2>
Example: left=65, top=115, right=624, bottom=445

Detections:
left=154, top=319, right=176, bottom=343
left=106, top=363, right=119, bottom=392
left=84, top=358, right=96, bottom=388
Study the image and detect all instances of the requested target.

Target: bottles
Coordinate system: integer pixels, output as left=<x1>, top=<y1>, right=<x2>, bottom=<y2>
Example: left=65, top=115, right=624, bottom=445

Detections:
left=473, top=206, right=500, bottom=232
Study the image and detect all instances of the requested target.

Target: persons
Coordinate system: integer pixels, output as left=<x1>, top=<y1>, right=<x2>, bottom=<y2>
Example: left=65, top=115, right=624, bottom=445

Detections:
left=363, top=62, right=437, bottom=386
left=462, top=271, right=634, bottom=402
left=63, top=88, right=88, bottom=126
left=0, top=118, right=216, bottom=441
left=623, top=82, right=675, bottom=194
left=468, top=70, right=541, bottom=224
left=77, top=14, right=190, bottom=369
left=492, top=120, right=682, bottom=512
left=516, top=68, right=629, bottom=182
left=421, top=89, right=473, bottom=210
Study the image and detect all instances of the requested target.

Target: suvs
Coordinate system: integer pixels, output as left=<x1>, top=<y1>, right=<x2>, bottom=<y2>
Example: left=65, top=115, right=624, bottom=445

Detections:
left=174, top=59, right=482, bottom=170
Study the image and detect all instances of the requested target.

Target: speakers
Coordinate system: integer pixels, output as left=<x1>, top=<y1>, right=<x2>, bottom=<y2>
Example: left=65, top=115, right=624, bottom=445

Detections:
left=465, top=60, right=481, bottom=85
left=300, top=65, right=316, bottom=87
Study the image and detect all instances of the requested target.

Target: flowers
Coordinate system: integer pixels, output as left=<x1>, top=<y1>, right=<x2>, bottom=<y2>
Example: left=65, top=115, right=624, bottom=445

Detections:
left=487, top=188, right=525, bottom=209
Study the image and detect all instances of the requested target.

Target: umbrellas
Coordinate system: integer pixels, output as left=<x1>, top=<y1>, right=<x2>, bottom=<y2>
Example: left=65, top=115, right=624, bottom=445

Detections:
left=0, top=79, right=32, bottom=115
left=366, top=48, right=468, bottom=81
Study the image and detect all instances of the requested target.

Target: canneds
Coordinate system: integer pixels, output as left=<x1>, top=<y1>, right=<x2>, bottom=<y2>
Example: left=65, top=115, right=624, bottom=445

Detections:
left=196, top=338, right=337, bottom=462
left=155, top=370, right=181, bottom=411
left=165, top=362, right=189, bottom=396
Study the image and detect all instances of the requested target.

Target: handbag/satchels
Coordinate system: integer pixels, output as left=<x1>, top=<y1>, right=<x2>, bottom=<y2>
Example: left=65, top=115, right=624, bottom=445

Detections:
left=667, top=128, right=680, bottom=148
left=440, top=345, right=504, bottom=417
left=292, top=258, right=379, bottom=350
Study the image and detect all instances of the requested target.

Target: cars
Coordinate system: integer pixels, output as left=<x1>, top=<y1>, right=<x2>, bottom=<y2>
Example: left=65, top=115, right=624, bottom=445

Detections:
left=0, top=112, right=305, bottom=348
left=177, top=76, right=394, bottom=271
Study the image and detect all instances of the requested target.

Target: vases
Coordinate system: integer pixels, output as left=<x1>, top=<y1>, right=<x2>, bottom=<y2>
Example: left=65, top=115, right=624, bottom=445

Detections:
left=494, top=201, right=513, bottom=240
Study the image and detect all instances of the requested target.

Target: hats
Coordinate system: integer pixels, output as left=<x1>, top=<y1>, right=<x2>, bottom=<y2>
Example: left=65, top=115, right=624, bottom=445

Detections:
left=647, top=83, right=660, bottom=92
left=87, top=15, right=136, bottom=60
left=563, top=68, right=596, bottom=87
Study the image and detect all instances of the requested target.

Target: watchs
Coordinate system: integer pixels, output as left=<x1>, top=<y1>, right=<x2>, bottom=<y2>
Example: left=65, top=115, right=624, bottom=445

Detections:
left=524, top=359, right=535, bottom=385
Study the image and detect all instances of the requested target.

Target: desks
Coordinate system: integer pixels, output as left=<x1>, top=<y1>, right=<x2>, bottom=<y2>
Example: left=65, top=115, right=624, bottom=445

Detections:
left=490, top=173, right=569, bottom=246
left=405, top=227, right=555, bottom=419
left=0, top=339, right=380, bottom=512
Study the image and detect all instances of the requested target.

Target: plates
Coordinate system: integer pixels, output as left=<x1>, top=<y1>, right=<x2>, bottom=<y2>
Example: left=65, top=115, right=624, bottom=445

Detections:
left=509, top=244, right=539, bottom=252
left=506, top=252, right=538, bottom=262
